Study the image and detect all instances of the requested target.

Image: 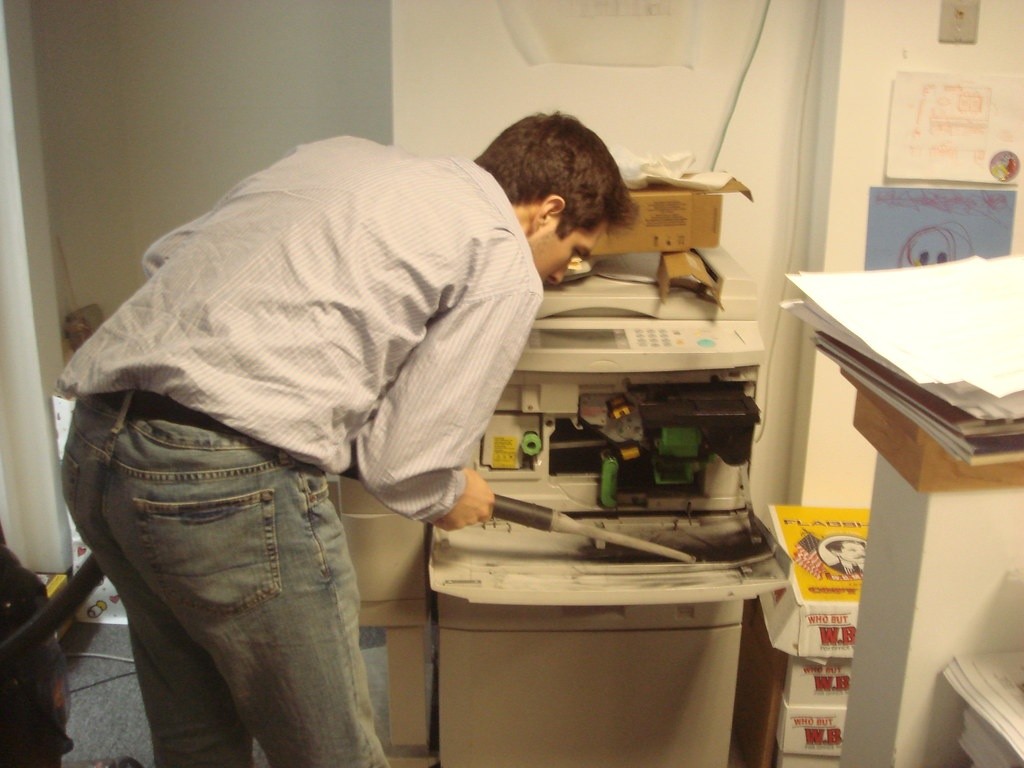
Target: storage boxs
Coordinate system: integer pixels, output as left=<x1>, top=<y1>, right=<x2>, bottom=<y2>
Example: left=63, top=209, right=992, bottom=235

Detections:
left=590, top=190, right=725, bottom=252
left=854, top=387, right=1024, bottom=493
left=734, top=506, right=871, bottom=767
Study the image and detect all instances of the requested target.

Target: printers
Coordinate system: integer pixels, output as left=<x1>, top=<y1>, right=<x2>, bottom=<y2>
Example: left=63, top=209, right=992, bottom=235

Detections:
left=426, top=247, right=789, bottom=612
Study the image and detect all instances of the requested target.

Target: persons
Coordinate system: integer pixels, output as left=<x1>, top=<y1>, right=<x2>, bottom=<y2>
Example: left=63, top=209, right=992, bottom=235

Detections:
left=61, top=111, right=635, bottom=768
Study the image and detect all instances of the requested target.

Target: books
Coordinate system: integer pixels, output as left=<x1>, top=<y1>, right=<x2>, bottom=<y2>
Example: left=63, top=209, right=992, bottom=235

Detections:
left=806, top=328, right=1024, bottom=468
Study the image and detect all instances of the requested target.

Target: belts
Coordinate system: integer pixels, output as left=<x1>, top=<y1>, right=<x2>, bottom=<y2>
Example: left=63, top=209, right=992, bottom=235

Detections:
left=94, top=387, right=241, bottom=435
